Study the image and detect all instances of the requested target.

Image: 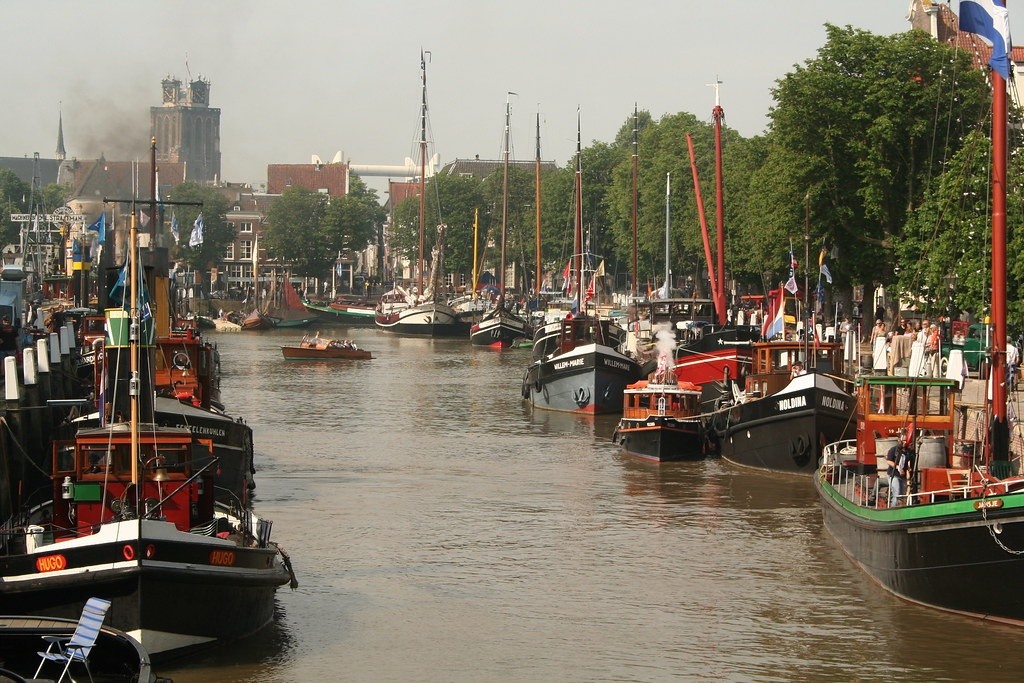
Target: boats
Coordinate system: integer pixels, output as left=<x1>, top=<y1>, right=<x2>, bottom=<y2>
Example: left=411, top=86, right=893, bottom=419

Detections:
left=709, top=192, right=858, bottom=479
left=280, top=337, right=371, bottom=361
left=0, top=613, right=153, bottom=683
left=611, top=363, right=705, bottom=465
left=0, top=132, right=292, bottom=660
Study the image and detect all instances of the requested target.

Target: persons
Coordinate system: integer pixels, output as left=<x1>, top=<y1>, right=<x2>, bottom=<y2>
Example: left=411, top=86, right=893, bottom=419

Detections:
left=302, top=333, right=309, bottom=342
left=870, top=319, right=1020, bottom=403
left=315, top=330, right=319, bottom=338
left=839, top=314, right=854, bottom=350
left=0, top=314, right=18, bottom=358
left=53, top=304, right=66, bottom=337
left=219, top=308, right=228, bottom=323
left=170, top=262, right=185, bottom=316
left=328, top=339, right=357, bottom=351
left=886, top=431, right=915, bottom=507
left=44, top=308, right=56, bottom=349
left=790, top=361, right=806, bottom=381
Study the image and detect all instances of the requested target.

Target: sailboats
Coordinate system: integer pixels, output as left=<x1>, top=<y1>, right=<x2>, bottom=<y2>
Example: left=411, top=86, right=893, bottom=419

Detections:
left=619, top=72, right=758, bottom=389
left=815, top=0, right=1024, bottom=627
left=526, top=105, right=633, bottom=416
left=302, top=46, right=548, bottom=346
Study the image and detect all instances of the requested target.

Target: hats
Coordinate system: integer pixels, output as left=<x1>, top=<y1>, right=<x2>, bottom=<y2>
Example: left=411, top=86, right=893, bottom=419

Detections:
left=792, top=361, right=803, bottom=367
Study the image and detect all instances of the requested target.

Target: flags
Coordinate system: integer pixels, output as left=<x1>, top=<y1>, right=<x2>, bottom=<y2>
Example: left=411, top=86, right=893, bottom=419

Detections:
left=819, top=250, right=832, bottom=283
left=593, top=260, right=604, bottom=277
left=170, top=212, right=180, bottom=241
left=189, top=213, right=204, bottom=252
left=140, top=209, right=150, bottom=227
left=138, top=252, right=151, bottom=311
left=583, top=277, right=594, bottom=304
left=109, top=255, right=129, bottom=308
left=762, top=250, right=800, bottom=339
left=72, top=237, right=80, bottom=254
left=87, top=210, right=105, bottom=245
left=562, top=261, right=572, bottom=289
left=571, top=290, right=577, bottom=317
